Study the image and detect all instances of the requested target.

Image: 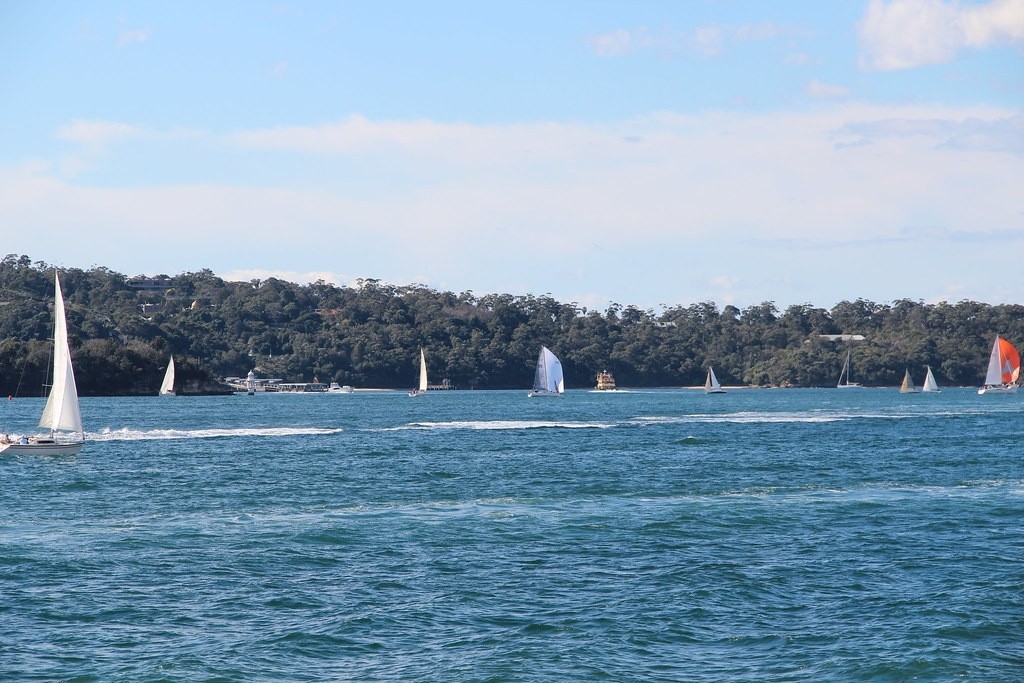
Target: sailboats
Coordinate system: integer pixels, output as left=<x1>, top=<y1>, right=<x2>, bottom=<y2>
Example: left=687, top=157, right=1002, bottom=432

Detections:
left=0, top=266, right=85, bottom=458
left=836, top=351, right=864, bottom=389
left=408, top=349, right=428, bottom=398
left=158, top=353, right=178, bottom=396
left=976, top=332, right=1022, bottom=396
left=704, top=367, right=728, bottom=394
left=923, top=363, right=941, bottom=394
left=898, top=367, right=919, bottom=393
left=527, top=346, right=566, bottom=399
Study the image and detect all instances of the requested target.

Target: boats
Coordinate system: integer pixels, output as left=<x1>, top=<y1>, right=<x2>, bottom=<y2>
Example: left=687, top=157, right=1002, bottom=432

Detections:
left=595, top=369, right=618, bottom=391
left=327, top=379, right=355, bottom=394
